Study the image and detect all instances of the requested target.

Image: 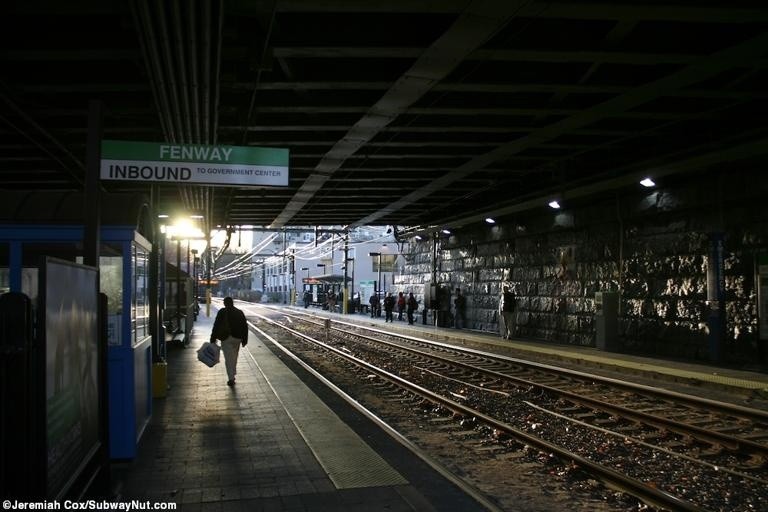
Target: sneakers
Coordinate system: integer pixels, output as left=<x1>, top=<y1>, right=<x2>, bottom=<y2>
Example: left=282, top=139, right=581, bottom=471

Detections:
left=227, top=377, right=236, bottom=387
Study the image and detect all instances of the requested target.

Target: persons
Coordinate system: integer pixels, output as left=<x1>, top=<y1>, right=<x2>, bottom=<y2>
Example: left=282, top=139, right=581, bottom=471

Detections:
left=302, top=290, right=312, bottom=307
left=453, top=286, right=467, bottom=327
left=369, top=291, right=416, bottom=325
left=499, top=286, right=515, bottom=339
left=327, top=288, right=338, bottom=314
left=210, top=296, right=248, bottom=386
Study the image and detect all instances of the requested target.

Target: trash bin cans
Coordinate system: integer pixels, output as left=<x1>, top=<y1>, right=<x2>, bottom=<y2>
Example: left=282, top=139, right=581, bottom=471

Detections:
left=348, top=300, right=355, bottom=313
left=262, top=295, right=267, bottom=302
left=153, top=364, right=167, bottom=397
left=434, top=310, right=444, bottom=328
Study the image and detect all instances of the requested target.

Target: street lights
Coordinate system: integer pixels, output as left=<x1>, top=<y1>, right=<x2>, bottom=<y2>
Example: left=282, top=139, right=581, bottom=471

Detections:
left=347, top=257, right=354, bottom=300
left=288, top=254, right=294, bottom=306
left=191, top=249, right=200, bottom=311
left=302, top=268, right=309, bottom=277
left=368, top=252, right=381, bottom=308
left=317, top=263, right=325, bottom=275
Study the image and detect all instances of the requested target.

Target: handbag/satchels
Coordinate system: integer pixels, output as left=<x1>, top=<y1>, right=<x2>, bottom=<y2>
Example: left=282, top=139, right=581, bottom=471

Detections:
left=195, top=340, right=222, bottom=369
left=215, top=317, right=229, bottom=342
